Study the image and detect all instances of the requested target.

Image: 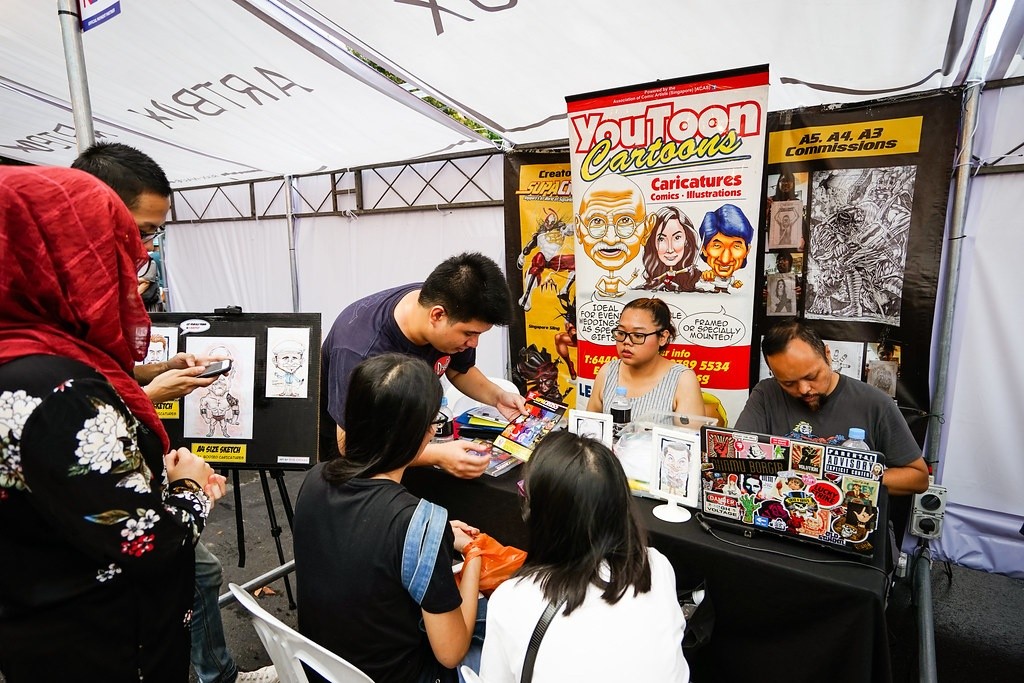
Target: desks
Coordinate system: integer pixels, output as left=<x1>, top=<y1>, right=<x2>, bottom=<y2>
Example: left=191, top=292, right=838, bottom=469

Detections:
left=403, top=446, right=890, bottom=683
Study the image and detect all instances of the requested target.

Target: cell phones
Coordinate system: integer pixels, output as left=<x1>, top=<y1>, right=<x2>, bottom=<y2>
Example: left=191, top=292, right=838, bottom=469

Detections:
left=194, top=360, right=232, bottom=378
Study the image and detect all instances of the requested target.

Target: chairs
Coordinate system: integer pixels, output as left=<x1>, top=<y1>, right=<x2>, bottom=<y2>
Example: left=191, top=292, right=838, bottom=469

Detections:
left=228, top=581, right=372, bottom=683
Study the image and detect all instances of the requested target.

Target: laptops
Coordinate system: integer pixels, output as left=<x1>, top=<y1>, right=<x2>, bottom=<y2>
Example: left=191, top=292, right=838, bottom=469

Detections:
left=701, top=425, right=886, bottom=561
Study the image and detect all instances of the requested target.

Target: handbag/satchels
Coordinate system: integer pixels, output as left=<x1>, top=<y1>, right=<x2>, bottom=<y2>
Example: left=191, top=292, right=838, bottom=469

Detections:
left=453, top=533, right=528, bottom=599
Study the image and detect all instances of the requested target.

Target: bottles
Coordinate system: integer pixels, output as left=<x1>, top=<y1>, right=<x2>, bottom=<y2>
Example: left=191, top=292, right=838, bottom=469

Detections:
left=431, top=398, right=454, bottom=469
left=611, top=388, right=632, bottom=450
left=842, top=428, right=870, bottom=451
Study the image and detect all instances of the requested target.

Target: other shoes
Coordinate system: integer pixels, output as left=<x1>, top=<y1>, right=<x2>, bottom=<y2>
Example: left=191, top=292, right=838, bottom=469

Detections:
left=235, top=664, right=278, bottom=683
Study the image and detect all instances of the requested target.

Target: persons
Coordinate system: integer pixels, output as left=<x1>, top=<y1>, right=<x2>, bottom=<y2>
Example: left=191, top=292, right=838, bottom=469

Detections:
left=763, top=253, right=801, bottom=310
left=0, top=165, right=227, bottom=683
left=865, top=343, right=901, bottom=379
left=70, top=141, right=238, bottom=683
left=318, top=252, right=530, bottom=479
left=767, top=174, right=805, bottom=252
left=733, top=317, right=929, bottom=497
left=480, top=430, right=690, bottom=683
left=586, top=298, right=705, bottom=453
left=294, top=352, right=482, bottom=683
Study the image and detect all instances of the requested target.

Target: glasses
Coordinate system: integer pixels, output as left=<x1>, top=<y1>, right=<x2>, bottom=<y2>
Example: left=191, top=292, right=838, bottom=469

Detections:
left=514, top=479, right=527, bottom=497
left=139, top=226, right=166, bottom=243
left=611, top=325, right=662, bottom=345
